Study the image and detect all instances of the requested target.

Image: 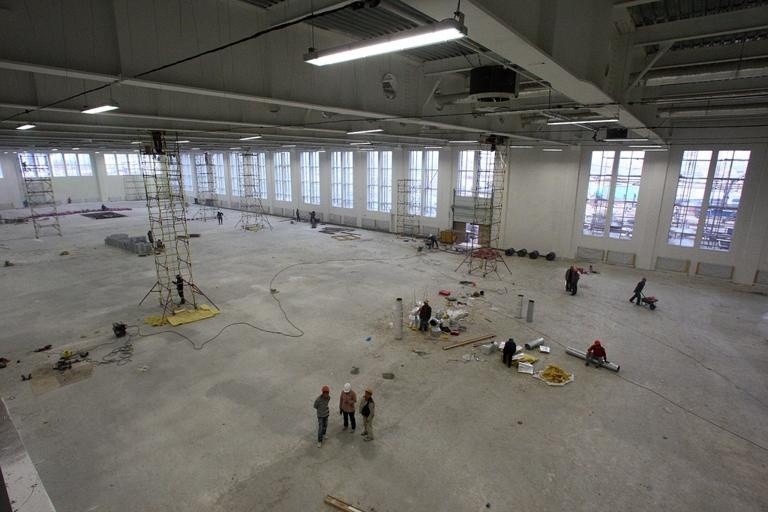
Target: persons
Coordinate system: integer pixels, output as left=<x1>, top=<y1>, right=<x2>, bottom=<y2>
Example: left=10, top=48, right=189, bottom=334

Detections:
left=296, top=208, right=300, bottom=222
left=314, top=385, right=331, bottom=448
left=217, top=212, right=224, bottom=225
left=427, top=234, right=439, bottom=248
left=172, top=275, right=185, bottom=306
left=339, top=383, right=357, bottom=433
left=630, top=277, right=647, bottom=305
left=502, top=338, right=516, bottom=369
left=419, top=299, right=432, bottom=331
left=565, top=265, right=580, bottom=295
left=359, top=388, right=375, bottom=441
left=585, top=339, right=610, bottom=368
left=310, top=211, right=316, bottom=224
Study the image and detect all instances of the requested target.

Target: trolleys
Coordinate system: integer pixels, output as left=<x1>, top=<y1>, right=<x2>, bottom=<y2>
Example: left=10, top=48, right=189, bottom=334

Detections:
left=636, top=289, right=661, bottom=311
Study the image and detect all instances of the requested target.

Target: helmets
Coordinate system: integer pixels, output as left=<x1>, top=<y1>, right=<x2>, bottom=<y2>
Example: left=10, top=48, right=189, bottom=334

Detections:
left=424, top=300, right=429, bottom=303
left=344, top=383, right=351, bottom=393
left=366, top=388, right=374, bottom=394
left=570, top=266, right=576, bottom=271
left=593, top=340, right=600, bottom=345
left=322, top=385, right=330, bottom=392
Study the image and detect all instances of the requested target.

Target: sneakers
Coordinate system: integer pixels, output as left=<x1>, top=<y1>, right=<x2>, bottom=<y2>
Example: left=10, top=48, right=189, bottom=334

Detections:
left=342, top=427, right=355, bottom=433
left=418, top=328, right=429, bottom=331
left=318, top=435, right=328, bottom=447
left=360, top=431, right=372, bottom=441
left=565, top=289, right=577, bottom=296
left=629, top=300, right=641, bottom=304
